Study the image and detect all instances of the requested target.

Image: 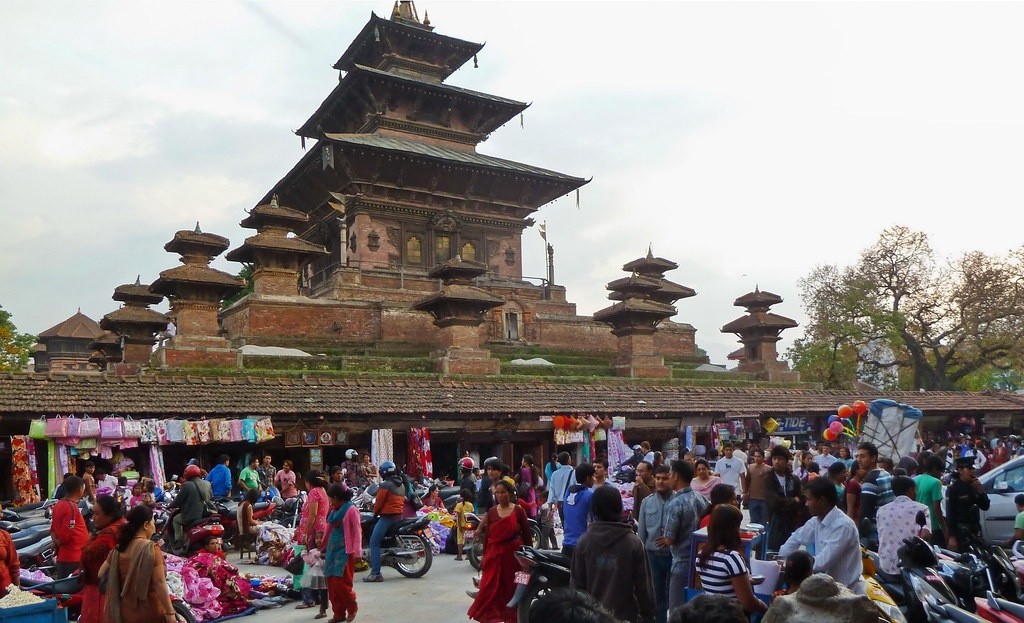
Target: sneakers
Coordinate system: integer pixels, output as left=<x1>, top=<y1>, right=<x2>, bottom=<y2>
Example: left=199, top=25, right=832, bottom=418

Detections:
left=362, top=573, right=384, bottom=582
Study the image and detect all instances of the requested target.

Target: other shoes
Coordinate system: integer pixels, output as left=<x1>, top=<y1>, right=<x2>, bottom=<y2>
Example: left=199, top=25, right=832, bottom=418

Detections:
left=174, top=541, right=185, bottom=549
left=346, top=609, right=357, bottom=622
left=314, top=612, right=327, bottom=619
left=295, top=602, right=315, bottom=609
left=328, top=616, right=347, bottom=623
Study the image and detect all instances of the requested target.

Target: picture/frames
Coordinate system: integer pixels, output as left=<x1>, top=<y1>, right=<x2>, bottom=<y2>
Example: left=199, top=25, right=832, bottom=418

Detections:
left=319, top=429, right=334, bottom=445
left=285, top=428, right=301, bottom=447
left=302, top=428, right=319, bottom=447
left=335, top=426, right=349, bottom=445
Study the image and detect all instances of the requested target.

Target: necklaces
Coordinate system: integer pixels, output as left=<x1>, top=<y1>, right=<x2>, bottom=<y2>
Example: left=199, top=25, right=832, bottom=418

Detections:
left=132, top=536, right=146, bottom=539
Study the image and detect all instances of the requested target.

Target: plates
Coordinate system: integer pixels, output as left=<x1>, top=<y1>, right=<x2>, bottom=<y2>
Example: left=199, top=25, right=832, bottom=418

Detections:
left=739, top=523, right=765, bottom=538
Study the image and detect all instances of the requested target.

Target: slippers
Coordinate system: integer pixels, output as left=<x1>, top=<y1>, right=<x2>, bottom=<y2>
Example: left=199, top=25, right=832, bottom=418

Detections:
left=454, top=555, right=463, bottom=561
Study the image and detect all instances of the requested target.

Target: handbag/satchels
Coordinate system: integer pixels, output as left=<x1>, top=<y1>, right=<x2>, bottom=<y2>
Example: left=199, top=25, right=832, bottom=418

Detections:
left=517, top=482, right=530, bottom=498
left=203, top=502, right=218, bottom=517
left=286, top=554, right=304, bottom=575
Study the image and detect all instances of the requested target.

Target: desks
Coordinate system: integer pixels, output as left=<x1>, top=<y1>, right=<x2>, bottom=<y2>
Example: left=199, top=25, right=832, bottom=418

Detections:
left=688, top=525, right=768, bottom=588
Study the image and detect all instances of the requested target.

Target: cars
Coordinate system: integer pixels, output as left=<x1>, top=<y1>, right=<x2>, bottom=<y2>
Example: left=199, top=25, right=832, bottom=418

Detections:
left=976, top=454, right=1024, bottom=548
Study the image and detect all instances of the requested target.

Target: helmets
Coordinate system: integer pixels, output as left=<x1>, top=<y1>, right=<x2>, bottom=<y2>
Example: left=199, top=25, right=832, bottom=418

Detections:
left=632, top=444, right=642, bottom=454
left=184, top=465, right=201, bottom=480
left=344, top=449, right=358, bottom=460
left=458, top=457, right=473, bottom=470
left=379, top=461, right=395, bottom=478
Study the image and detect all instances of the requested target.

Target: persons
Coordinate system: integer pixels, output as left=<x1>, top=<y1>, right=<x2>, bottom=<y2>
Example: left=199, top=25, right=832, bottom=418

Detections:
left=0, top=449, right=454, bottom=623
left=454, top=428, right=1024, bottom=623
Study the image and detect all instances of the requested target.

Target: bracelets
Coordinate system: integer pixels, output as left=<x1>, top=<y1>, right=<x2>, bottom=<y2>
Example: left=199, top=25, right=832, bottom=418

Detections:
left=302, top=531, right=308, bottom=537
left=165, top=612, right=176, bottom=615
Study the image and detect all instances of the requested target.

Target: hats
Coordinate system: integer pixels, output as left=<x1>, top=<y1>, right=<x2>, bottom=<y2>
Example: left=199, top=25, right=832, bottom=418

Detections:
left=187, top=458, right=199, bottom=466
left=955, top=455, right=976, bottom=469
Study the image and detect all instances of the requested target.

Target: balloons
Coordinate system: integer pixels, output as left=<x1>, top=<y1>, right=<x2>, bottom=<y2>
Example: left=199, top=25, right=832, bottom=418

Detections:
left=824, top=401, right=866, bottom=441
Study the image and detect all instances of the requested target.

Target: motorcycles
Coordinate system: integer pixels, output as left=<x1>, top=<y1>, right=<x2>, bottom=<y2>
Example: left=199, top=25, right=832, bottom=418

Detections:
left=463, top=490, right=544, bottom=571
left=351, top=500, right=434, bottom=579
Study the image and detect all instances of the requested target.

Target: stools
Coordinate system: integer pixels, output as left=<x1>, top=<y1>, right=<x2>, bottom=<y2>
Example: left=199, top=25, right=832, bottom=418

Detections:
left=240, top=533, right=258, bottom=561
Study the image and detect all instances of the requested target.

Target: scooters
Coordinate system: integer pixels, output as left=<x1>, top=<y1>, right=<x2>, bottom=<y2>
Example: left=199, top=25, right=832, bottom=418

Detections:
left=0, top=493, right=128, bottom=584
left=940, top=453, right=959, bottom=487
left=151, top=480, right=307, bottom=557
left=349, top=464, right=462, bottom=514
left=860, top=509, right=1024, bottom=623
left=612, top=460, right=636, bottom=485
